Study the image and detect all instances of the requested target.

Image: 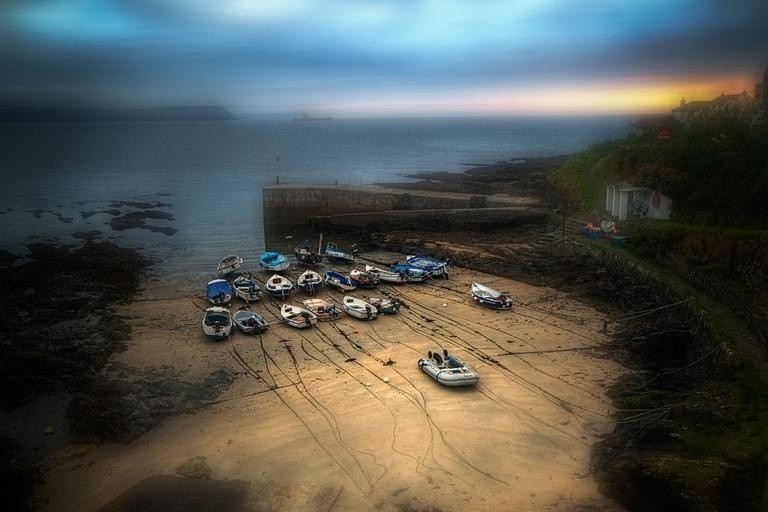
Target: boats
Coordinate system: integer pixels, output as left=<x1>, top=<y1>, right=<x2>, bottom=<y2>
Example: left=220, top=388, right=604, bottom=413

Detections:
left=469, top=282, right=514, bottom=310
left=202, top=242, right=450, bottom=341
left=418, top=354, right=480, bottom=387
